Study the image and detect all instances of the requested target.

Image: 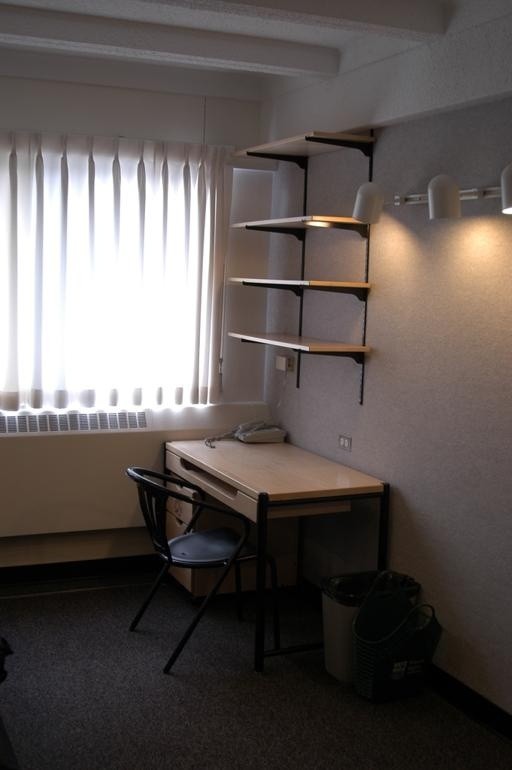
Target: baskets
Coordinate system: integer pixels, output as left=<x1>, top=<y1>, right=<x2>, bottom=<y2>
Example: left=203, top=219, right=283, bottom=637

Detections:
left=351, top=569, right=442, bottom=705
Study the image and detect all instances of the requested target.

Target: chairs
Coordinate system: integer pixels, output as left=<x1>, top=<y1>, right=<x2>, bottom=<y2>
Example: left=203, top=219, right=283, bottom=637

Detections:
left=126, top=467, right=281, bottom=674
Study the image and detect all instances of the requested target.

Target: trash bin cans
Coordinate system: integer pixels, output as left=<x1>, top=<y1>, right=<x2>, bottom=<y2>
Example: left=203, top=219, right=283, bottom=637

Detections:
left=322, top=570, right=420, bottom=682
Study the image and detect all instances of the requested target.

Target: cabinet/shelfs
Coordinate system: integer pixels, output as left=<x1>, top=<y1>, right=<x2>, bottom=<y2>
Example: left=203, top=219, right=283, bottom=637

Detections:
left=226, top=130, right=379, bottom=404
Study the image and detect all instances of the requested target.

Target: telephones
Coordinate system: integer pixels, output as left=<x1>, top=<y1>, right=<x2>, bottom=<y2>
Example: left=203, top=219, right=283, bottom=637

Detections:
left=234, top=419, right=287, bottom=443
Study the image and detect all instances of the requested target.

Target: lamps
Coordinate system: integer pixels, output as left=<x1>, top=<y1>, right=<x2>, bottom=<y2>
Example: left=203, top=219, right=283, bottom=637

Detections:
left=350, top=164, right=511, bottom=226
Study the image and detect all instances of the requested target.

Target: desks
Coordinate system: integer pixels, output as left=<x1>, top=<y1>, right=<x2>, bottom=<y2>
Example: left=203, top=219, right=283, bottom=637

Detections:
left=160, top=438, right=391, bottom=672
